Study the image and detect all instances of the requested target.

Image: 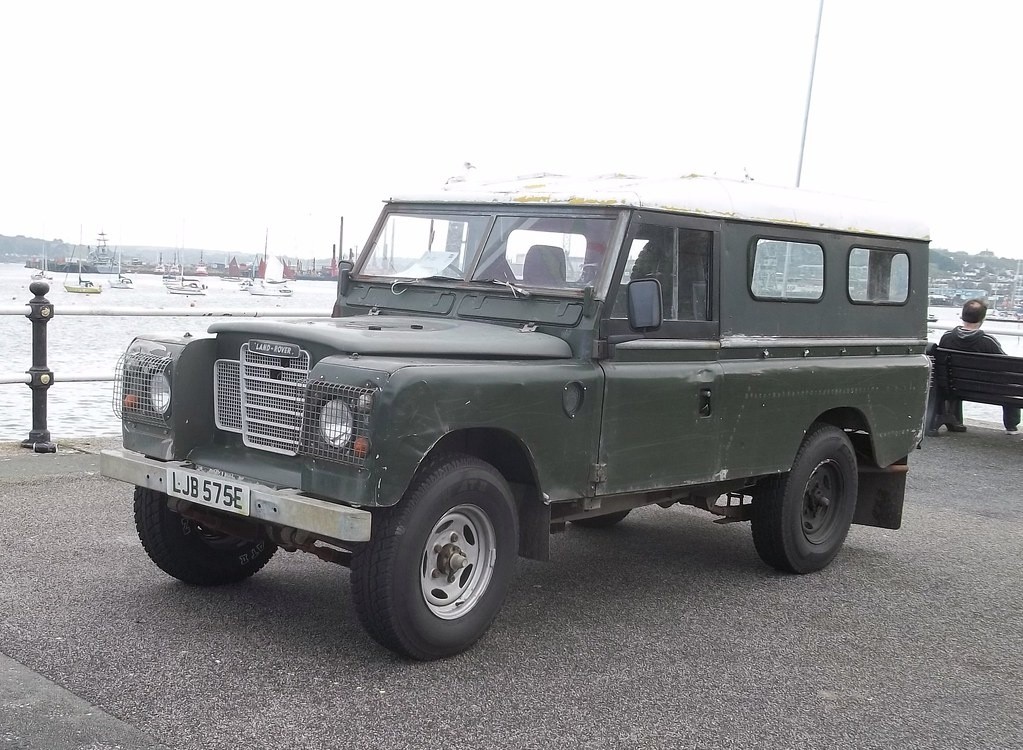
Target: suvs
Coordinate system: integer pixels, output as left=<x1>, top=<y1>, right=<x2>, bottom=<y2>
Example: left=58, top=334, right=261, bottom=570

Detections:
left=96, top=198, right=934, bottom=663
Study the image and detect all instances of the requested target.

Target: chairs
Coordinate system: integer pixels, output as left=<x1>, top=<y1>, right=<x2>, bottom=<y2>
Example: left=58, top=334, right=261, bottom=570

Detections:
left=522, top=244, right=567, bottom=288
left=655, top=250, right=704, bottom=320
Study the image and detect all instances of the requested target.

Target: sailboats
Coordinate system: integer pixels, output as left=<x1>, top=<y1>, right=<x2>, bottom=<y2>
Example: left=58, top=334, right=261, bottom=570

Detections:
left=63, top=223, right=103, bottom=294
left=986, top=261, right=1023, bottom=321
left=108, top=249, right=137, bottom=288
left=31, top=241, right=53, bottom=281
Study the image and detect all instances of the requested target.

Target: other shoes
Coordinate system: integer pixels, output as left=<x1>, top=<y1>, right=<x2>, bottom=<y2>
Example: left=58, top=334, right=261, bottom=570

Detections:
left=1005, top=427, right=1019, bottom=435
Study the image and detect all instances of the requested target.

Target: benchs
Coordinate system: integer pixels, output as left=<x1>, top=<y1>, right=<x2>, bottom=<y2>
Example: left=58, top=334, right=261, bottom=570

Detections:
left=925, top=342, right=1023, bottom=438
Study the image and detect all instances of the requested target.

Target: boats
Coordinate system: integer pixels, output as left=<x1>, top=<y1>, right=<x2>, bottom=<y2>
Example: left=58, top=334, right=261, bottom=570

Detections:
left=162, top=264, right=209, bottom=295
left=220, top=226, right=294, bottom=297
left=93, top=229, right=121, bottom=275
left=926, top=314, right=939, bottom=323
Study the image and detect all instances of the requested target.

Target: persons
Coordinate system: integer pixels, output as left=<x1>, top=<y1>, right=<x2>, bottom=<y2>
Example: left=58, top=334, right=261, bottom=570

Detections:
left=938, top=298, right=1020, bottom=435
